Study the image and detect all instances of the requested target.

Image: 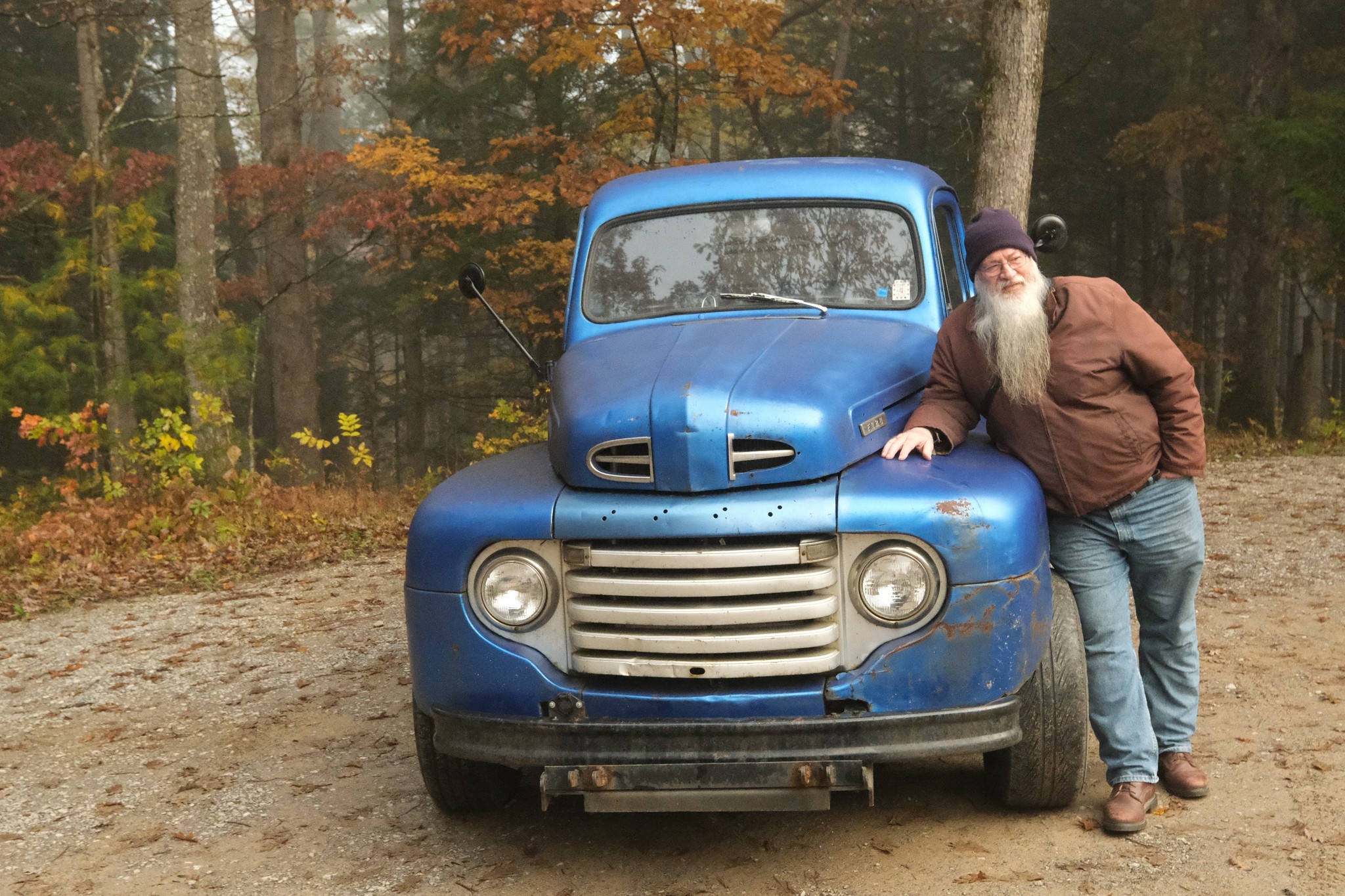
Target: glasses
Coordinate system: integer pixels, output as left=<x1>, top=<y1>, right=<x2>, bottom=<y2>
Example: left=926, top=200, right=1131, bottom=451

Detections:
left=983, top=249, right=1028, bottom=284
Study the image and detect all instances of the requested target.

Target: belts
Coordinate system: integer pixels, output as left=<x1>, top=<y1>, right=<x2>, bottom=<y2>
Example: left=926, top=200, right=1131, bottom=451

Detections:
left=1111, top=468, right=1161, bottom=509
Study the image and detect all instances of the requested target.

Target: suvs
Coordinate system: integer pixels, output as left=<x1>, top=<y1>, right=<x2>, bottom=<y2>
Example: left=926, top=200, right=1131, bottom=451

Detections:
left=403, top=154, right=1091, bottom=820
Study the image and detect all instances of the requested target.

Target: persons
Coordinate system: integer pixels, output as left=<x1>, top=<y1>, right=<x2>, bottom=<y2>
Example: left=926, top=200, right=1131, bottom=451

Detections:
left=881, top=207, right=1209, bottom=832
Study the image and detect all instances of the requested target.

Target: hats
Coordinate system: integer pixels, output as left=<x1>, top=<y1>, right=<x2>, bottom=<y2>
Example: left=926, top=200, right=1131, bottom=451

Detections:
left=963, top=208, right=1039, bottom=283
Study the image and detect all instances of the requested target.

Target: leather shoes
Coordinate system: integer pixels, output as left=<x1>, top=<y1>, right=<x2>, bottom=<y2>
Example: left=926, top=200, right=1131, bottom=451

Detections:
left=1158, top=749, right=1209, bottom=799
left=1101, top=780, right=1156, bottom=834
